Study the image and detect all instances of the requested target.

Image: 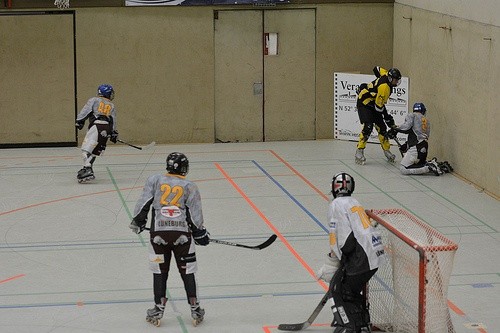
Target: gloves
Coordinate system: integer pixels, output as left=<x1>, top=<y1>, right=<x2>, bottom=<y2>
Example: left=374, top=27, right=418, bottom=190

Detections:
left=399, top=144, right=409, bottom=153
left=385, top=115, right=394, bottom=127
left=131, top=219, right=146, bottom=234
left=192, top=226, right=209, bottom=245
left=387, top=128, right=398, bottom=139
left=110, top=130, right=118, bottom=143
left=76, top=122, right=85, bottom=130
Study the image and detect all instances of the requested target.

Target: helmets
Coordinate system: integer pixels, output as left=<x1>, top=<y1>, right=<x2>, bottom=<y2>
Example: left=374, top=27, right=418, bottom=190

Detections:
left=413, top=103, right=426, bottom=115
left=388, top=68, right=401, bottom=87
left=97, top=84, right=114, bottom=101
left=167, top=152, right=188, bottom=175
left=332, top=173, right=354, bottom=199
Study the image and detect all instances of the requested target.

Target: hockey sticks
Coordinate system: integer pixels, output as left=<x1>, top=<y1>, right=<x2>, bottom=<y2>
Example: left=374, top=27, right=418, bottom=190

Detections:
left=371, top=124, right=407, bottom=153
left=277, top=288, right=330, bottom=331
left=384, top=112, right=405, bottom=157
left=143, top=226, right=278, bottom=251
left=107, top=135, right=156, bottom=151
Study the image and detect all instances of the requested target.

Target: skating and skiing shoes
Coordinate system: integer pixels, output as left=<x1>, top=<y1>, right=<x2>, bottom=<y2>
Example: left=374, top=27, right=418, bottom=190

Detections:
left=190, top=303, right=205, bottom=326
left=146, top=297, right=168, bottom=326
left=77, top=167, right=95, bottom=183
left=355, top=150, right=366, bottom=165
left=385, top=150, right=395, bottom=163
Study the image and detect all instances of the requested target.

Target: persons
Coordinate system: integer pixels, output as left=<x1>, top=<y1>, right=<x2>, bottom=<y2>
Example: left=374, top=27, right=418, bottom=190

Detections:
left=74, top=83, right=118, bottom=183
left=393, top=103, right=454, bottom=176
left=355, top=65, right=401, bottom=164
left=328, top=172, right=386, bottom=333
left=130, top=151, right=211, bottom=327
